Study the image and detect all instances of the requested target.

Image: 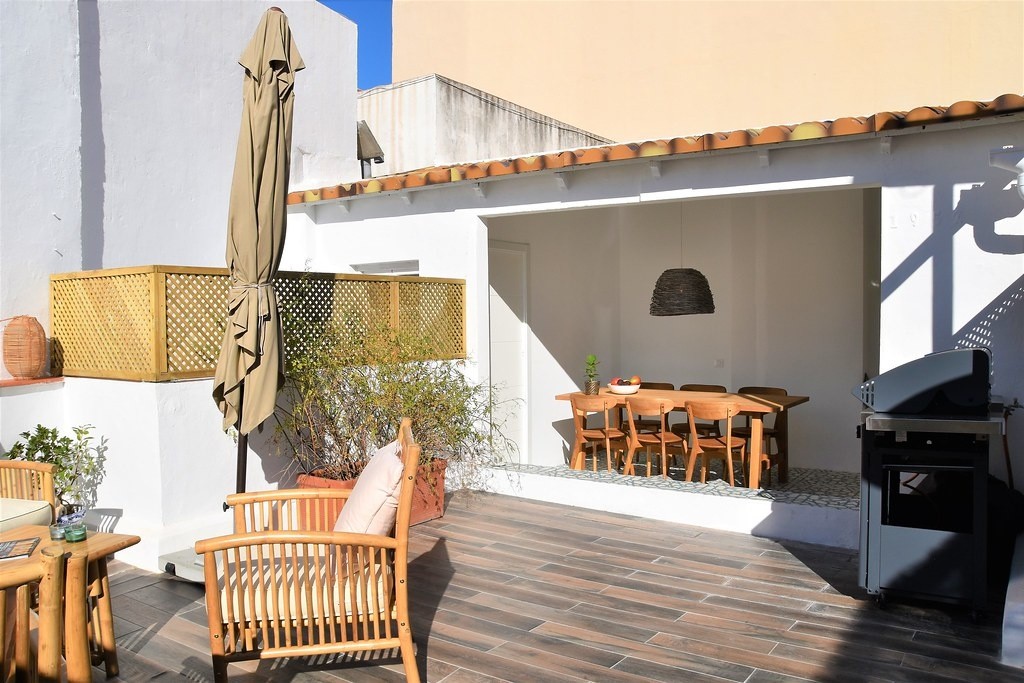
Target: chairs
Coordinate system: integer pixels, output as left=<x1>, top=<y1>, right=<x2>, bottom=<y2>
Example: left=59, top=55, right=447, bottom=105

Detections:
left=569, top=393, right=635, bottom=476
left=724, top=386, right=787, bottom=488
left=193, top=417, right=420, bottom=683
left=684, top=398, right=749, bottom=487
left=621, top=382, right=677, bottom=474
left=666, top=384, right=727, bottom=481
left=0, top=460, right=58, bottom=525
left=1, top=544, right=63, bottom=683
left=623, top=396, right=689, bottom=477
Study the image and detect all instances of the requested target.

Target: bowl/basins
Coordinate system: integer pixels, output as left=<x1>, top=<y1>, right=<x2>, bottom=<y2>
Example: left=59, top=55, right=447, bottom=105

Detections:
left=607, top=383, right=640, bottom=394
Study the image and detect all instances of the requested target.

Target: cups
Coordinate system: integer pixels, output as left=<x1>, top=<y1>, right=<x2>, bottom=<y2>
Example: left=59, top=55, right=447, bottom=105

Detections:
left=65, top=525, right=87, bottom=542
left=49, top=523, right=70, bottom=540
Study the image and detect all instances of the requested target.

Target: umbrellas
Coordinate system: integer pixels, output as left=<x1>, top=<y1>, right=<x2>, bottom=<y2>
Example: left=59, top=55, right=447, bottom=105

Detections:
left=212, top=6, right=305, bottom=496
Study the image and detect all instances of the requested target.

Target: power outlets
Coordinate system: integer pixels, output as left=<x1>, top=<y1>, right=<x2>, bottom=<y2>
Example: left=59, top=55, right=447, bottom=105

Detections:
left=713, top=359, right=724, bottom=369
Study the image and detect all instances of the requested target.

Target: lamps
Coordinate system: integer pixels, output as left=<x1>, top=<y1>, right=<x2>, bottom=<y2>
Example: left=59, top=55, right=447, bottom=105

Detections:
left=649, top=202, right=716, bottom=316
left=758, top=150, right=769, bottom=167
left=649, top=162, right=662, bottom=180
left=471, top=183, right=487, bottom=198
left=400, top=192, right=412, bottom=206
left=338, top=201, right=349, bottom=215
left=879, top=137, right=892, bottom=156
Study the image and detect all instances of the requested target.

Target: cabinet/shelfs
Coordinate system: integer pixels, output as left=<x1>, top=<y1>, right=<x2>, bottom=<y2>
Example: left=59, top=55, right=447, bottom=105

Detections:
left=858, top=407, right=1005, bottom=613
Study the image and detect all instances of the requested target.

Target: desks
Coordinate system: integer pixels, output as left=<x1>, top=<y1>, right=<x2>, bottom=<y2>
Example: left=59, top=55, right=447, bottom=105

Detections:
left=0, top=525, right=142, bottom=683
left=555, top=387, right=810, bottom=489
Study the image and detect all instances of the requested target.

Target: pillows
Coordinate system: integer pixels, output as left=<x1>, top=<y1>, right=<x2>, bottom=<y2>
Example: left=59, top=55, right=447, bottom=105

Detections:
left=330, top=440, right=404, bottom=581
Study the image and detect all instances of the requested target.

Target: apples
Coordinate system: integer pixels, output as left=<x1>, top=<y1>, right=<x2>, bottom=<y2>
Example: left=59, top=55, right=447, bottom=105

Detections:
left=611, top=375, right=640, bottom=385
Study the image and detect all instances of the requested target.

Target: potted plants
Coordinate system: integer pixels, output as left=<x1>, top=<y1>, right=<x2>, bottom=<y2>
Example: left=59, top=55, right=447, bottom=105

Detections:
left=583, top=354, right=601, bottom=395
left=272, top=303, right=525, bottom=531
left=2, top=423, right=98, bottom=528
